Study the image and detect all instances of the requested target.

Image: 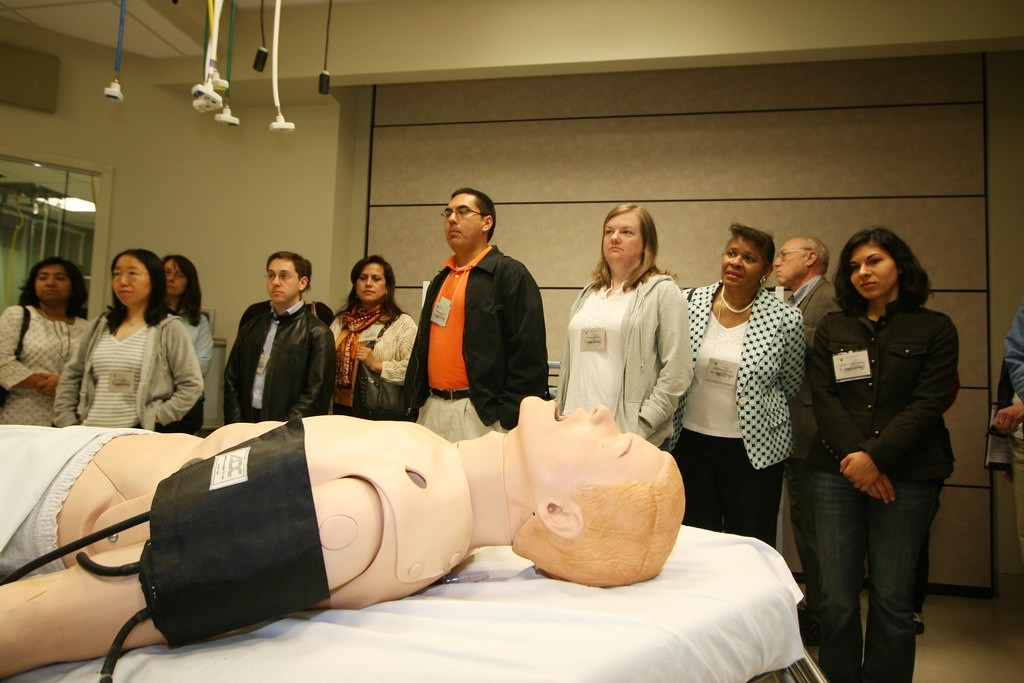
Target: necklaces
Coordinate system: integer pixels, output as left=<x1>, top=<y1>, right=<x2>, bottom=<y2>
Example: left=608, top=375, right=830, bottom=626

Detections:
left=126, top=318, right=145, bottom=326
left=38, top=304, right=70, bottom=358
left=722, top=285, right=756, bottom=314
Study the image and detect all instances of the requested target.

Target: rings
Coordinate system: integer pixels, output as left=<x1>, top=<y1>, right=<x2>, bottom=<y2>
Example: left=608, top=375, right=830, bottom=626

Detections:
left=998, top=420, right=1003, bottom=425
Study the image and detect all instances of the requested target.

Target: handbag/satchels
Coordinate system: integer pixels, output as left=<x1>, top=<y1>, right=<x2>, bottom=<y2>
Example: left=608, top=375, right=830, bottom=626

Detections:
left=0, top=306, right=29, bottom=410
left=352, top=312, right=415, bottom=420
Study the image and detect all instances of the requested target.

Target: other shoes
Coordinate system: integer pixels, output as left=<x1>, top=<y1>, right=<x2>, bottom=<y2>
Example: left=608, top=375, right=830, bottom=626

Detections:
left=915, top=621, right=924, bottom=634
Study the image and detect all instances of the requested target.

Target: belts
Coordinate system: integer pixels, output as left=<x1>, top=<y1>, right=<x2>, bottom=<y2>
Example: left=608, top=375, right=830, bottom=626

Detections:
left=427, top=386, right=470, bottom=400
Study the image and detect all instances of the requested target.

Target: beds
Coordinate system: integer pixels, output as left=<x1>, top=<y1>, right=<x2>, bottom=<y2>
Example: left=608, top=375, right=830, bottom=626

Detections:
left=1, top=524, right=829, bottom=683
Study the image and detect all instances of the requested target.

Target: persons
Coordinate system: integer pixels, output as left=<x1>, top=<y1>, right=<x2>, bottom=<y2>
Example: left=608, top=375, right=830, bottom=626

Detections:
left=554, top=203, right=694, bottom=454
left=223, top=251, right=337, bottom=425
left=810, top=227, right=958, bottom=683
left=404, top=188, right=550, bottom=444
left=155, top=254, right=214, bottom=435
left=0, top=396, right=685, bottom=683
left=53, top=248, right=204, bottom=430
left=774, top=236, right=843, bottom=646
left=680, top=223, right=804, bottom=548
left=330, top=255, right=418, bottom=421
left=991, top=295, right=1024, bottom=554
left=0, top=257, right=89, bottom=427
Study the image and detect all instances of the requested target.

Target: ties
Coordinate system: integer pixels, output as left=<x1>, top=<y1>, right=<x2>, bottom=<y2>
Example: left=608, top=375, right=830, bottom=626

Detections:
left=788, top=294, right=794, bottom=304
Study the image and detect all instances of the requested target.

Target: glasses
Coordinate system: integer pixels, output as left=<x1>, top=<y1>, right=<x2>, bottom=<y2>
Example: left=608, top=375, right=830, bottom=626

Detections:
left=441, top=208, right=486, bottom=217
left=777, top=250, right=812, bottom=260
